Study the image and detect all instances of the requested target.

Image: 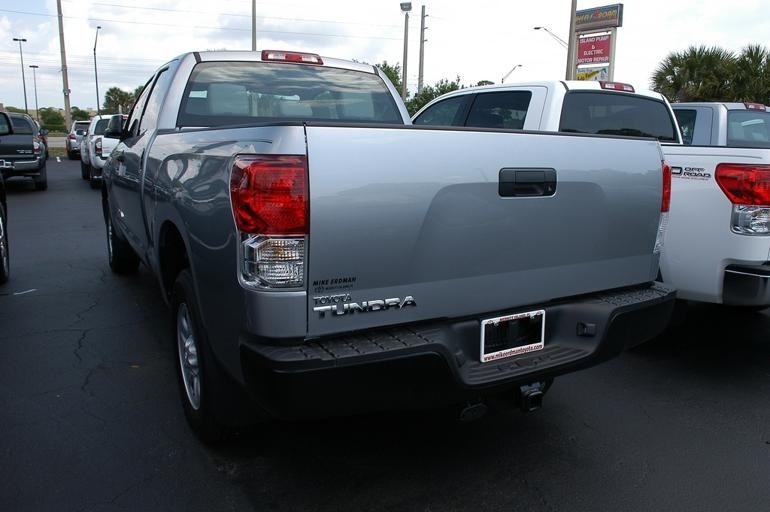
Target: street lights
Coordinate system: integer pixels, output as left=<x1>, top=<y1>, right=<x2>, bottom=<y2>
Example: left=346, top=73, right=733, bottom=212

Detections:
left=13, top=38, right=29, bottom=114
left=533, top=27, right=569, bottom=50
left=400, top=2, right=412, bottom=101
left=29, top=65, right=39, bottom=121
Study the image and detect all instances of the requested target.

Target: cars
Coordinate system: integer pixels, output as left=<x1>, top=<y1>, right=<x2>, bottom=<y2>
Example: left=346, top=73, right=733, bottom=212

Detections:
left=0, top=113, right=49, bottom=281
